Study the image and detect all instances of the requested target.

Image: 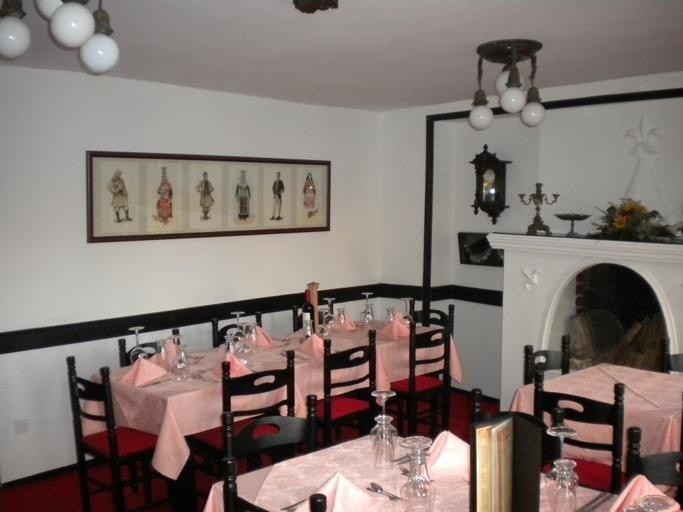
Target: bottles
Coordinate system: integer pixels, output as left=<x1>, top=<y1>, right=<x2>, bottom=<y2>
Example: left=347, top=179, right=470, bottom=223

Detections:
left=301, top=290, right=314, bottom=341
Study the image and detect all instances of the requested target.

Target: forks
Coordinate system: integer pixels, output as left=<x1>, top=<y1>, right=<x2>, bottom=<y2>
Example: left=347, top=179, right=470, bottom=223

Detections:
left=366, top=486, right=397, bottom=501
left=399, top=465, right=411, bottom=480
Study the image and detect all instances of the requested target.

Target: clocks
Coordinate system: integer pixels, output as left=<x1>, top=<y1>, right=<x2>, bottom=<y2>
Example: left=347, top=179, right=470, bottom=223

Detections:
left=471, top=146, right=511, bottom=223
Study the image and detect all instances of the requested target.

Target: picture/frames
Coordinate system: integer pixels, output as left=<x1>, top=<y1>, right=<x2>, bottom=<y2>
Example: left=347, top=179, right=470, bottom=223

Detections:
left=85, top=149, right=335, bottom=245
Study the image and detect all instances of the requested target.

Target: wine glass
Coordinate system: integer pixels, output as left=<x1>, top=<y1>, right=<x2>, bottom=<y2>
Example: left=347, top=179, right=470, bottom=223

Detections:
left=322, top=297, right=336, bottom=332
left=224, top=335, right=234, bottom=353
left=170, top=334, right=189, bottom=380
left=128, top=326, right=147, bottom=365
left=399, top=436, right=434, bottom=512
left=364, top=304, right=374, bottom=321
left=552, top=459, right=578, bottom=512
left=303, top=320, right=312, bottom=342
left=336, top=308, right=346, bottom=330
left=384, top=305, right=394, bottom=324
left=634, top=495, right=673, bottom=512
left=230, top=311, right=245, bottom=342
left=372, top=391, right=397, bottom=457
left=359, top=292, right=372, bottom=327
left=544, top=427, right=579, bottom=510
left=369, top=416, right=398, bottom=471
left=401, top=297, right=412, bottom=326
left=155, top=338, right=169, bottom=373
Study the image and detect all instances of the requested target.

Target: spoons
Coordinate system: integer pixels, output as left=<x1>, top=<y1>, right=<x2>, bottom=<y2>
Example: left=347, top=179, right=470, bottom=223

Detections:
left=368, top=483, right=402, bottom=502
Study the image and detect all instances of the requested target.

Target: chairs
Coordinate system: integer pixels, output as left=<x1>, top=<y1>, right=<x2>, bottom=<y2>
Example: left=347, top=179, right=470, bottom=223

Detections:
left=626, top=420, right=683, bottom=510
left=118, top=328, right=186, bottom=367
left=388, top=322, right=452, bottom=438
left=215, top=394, right=336, bottom=476
left=211, top=311, right=264, bottom=350
left=64, top=357, right=194, bottom=512
left=521, top=334, right=575, bottom=386
left=467, top=386, right=566, bottom=487
left=310, top=330, right=379, bottom=448
left=532, top=371, right=622, bottom=497
left=188, top=350, right=295, bottom=508
left=222, top=456, right=328, bottom=512
left=292, top=300, right=334, bottom=334
left=407, top=295, right=456, bottom=331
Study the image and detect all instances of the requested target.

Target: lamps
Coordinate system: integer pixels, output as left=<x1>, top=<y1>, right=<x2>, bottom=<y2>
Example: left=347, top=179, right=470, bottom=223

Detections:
left=467, top=38, right=550, bottom=131
left=0, top=0, right=124, bottom=78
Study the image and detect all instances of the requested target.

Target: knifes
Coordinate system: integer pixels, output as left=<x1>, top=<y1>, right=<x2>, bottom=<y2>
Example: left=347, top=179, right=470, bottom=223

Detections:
left=577, top=490, right=607, bottom=512
left=142, top=376, right=172, bottom=388
left=588, top=493, right=614, bottom=512
left=284, top=349, right=309, bottom=361
left=280, top=352, right=297, bottom=364
